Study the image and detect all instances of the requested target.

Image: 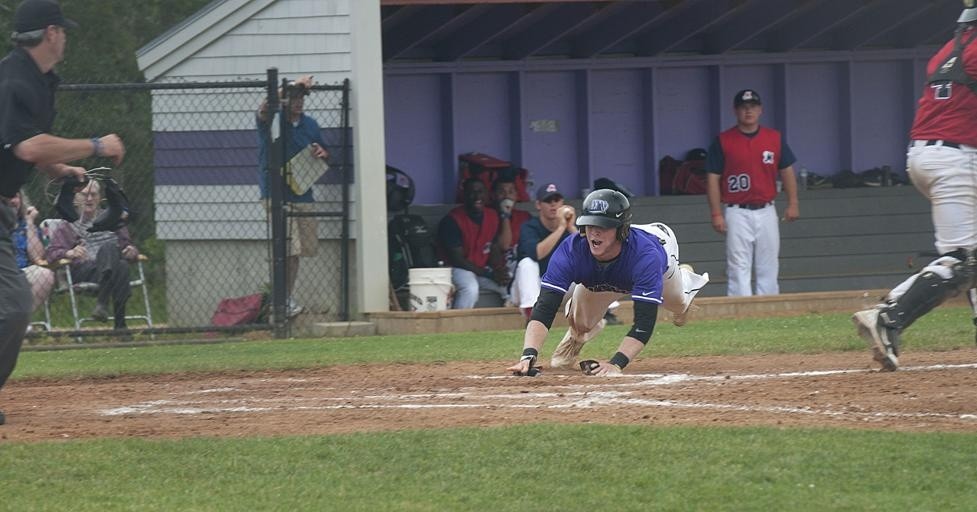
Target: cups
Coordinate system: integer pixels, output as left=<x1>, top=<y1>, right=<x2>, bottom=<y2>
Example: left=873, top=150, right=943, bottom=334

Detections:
left=776, top=181, right=784, bottom=192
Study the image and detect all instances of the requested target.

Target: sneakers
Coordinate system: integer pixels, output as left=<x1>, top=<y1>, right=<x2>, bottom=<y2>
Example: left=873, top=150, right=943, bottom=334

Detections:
left=852, top=303, right=901, bottom=371
left=550, top=326, right=585, bottom=370
left=91, top=303, right=109, bottom=323
left=807, top=172, right=834, bottom=190
left=270, top=302, right=305, bottom=328
left=112, top=323, right=135, bottom=342
left=672, top=264, right=695, bottom=326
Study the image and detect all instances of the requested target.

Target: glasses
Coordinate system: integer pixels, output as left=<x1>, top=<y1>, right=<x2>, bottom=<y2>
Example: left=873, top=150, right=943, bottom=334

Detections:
left=544, top=198, right=562, bottom=203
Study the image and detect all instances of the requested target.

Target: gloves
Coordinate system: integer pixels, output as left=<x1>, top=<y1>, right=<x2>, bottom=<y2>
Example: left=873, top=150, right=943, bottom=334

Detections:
left=500, top=199, right=515, bottom=217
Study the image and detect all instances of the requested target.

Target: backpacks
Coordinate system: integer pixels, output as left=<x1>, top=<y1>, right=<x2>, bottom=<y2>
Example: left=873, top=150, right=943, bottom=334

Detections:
left=387, top=215, right=445, bottom=293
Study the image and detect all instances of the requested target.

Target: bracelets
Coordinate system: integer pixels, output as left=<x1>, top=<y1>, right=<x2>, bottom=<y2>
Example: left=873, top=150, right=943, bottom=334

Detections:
left=714, top=212, right=721, bottom=218
left=607, top=352, right=630, bottom=372
left=89, top=136, right=103, bottom=159
left=519, top=347, right=538, bottom=362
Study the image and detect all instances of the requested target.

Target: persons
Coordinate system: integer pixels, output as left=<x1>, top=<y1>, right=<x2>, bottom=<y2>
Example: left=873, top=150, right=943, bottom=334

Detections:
left=8, top=191, right=57, bottom=343
left=508, top=182, right=626, bottom=332
left=503, top=188, right=713, bottom=375
left=43, top=173, right=141, bottom=346
left=438, top=178, right=512, bottom=311
left=850, top=1, right=977, bottom=373
left=0, top=0, right=128, bottom=427
left=488, top=178, right=532, bottom=279
left=253, top=73, right=330, bottom=327
left=704, top=86, right=800, bottom=297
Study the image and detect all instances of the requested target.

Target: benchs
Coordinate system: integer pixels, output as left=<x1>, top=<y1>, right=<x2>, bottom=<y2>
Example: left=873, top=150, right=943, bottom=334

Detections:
left=389, top=185, right=933, bottom=310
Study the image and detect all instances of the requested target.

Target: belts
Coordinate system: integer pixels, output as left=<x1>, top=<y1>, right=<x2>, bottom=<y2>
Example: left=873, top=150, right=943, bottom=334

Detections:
left=908, top=140, right=960, bottom=149
left=728, top=202, right=773, bottom=210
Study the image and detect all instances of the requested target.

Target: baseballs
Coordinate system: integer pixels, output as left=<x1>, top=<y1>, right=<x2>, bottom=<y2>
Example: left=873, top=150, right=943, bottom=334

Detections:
left=562, top=207, right=574, bottom=219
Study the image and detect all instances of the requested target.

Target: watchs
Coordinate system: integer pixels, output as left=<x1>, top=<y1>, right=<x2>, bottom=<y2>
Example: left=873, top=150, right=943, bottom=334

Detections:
left=498, top=212, right=513, bottom=220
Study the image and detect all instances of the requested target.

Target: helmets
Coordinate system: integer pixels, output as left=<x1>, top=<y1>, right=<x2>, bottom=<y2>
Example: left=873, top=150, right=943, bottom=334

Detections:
left=575, top=187, right=633, bottom=230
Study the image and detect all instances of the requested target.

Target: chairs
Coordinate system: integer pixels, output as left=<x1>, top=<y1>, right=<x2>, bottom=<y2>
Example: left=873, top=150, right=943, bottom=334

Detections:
left=17, top=219, right=156, bottom=344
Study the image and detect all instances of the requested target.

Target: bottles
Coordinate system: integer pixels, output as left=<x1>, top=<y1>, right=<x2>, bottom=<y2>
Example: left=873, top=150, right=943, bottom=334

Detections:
left=880, top=165, right=894, bottom=187
left=798, top=167, right=809, bottom=190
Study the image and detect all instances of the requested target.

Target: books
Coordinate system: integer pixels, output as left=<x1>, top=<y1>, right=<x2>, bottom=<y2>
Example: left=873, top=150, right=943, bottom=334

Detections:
left=279, top=145, right=329, bottom=196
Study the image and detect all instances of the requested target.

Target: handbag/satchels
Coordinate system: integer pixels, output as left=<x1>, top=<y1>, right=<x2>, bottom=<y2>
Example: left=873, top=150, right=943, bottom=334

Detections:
left=659, top=148, right=707, bottom=195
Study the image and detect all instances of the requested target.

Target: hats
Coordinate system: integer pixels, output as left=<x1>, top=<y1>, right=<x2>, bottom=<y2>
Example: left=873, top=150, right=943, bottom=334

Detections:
left=11, top=2, right=79, bottom=34
left=733, top=89, right=761, bottom=105
left=535, top=182, right=565, bottom=202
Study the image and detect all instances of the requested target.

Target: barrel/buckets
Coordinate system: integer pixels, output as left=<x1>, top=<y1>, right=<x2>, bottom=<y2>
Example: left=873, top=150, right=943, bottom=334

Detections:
left=406, top=266, right=454, bottom=313
